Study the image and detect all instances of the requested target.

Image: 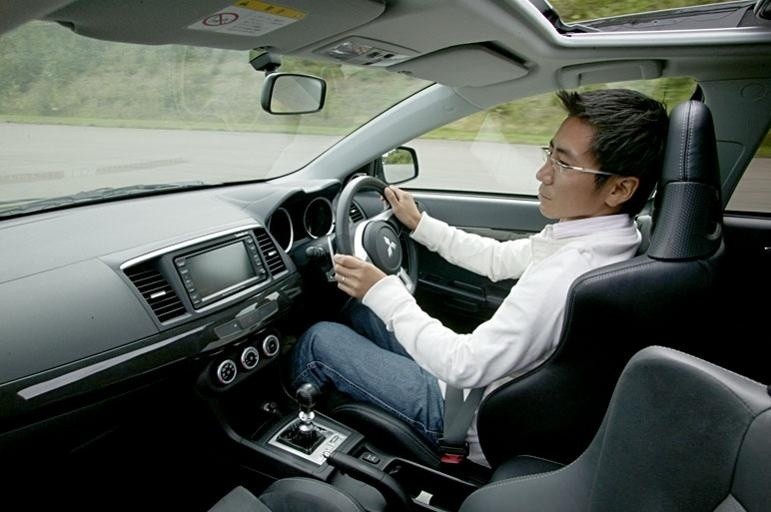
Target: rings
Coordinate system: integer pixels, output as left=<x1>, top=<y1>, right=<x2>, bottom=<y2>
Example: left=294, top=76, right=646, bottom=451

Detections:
left=342, top=275, right=346, bottom=284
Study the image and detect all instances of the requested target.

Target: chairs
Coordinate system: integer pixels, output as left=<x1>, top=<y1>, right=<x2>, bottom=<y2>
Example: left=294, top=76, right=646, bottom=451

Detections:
left=208, top=344, right=770, bottom=512
left=328, top=100, right=727, bottom=471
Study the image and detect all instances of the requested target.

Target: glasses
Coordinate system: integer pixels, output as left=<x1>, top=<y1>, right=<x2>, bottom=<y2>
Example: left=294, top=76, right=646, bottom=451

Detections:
left=539, top=146, right=620, bottom=178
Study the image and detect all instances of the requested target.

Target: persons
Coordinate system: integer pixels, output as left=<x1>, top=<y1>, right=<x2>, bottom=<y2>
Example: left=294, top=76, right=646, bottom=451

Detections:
left=277, top=87, right=673, bottom=471
